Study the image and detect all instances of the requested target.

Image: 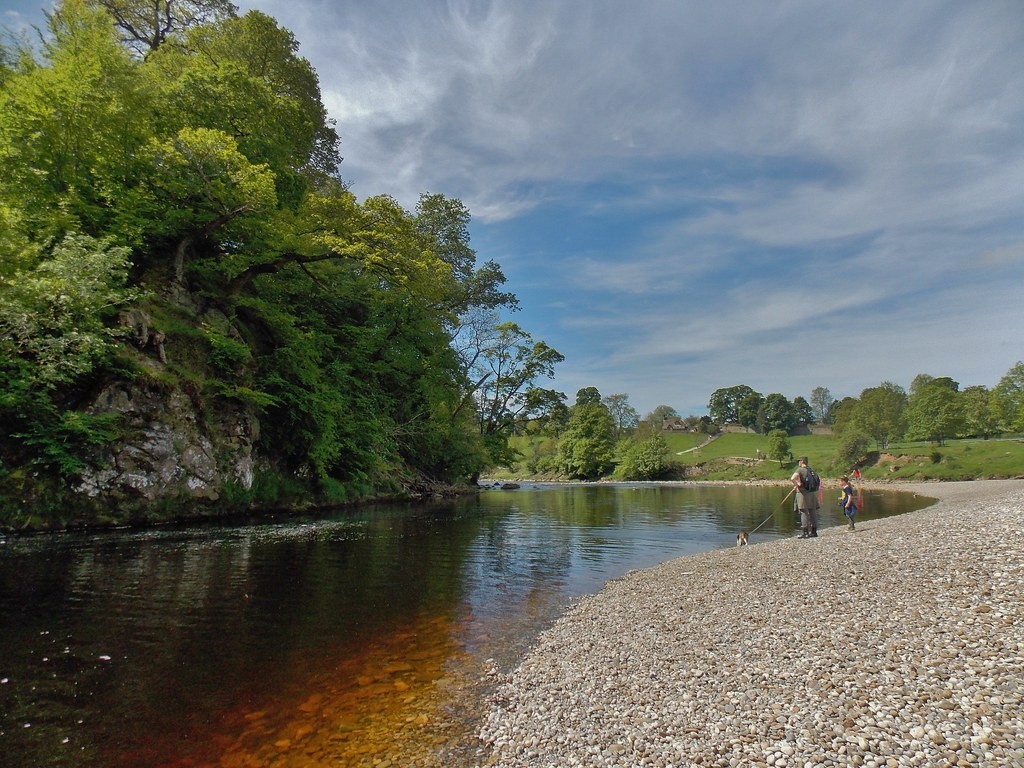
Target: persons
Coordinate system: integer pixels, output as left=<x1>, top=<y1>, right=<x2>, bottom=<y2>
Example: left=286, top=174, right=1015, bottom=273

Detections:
left=788, top=452, right=792, bottom=462
left=850, top=466, right=862, bottom=486
left=757, top=449, right=767, bottom=460
left=840, top=475, right=855, bottom=533
left=791, top=456, right=819, bottom=539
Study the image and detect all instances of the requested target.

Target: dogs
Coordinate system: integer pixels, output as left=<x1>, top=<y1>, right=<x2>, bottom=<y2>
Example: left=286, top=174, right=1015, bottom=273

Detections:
left=737, top=532, right=749, bottom=546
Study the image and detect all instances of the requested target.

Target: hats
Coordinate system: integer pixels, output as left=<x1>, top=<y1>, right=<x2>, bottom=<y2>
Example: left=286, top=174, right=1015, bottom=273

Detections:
left=797, top=456, right=808, bottom=460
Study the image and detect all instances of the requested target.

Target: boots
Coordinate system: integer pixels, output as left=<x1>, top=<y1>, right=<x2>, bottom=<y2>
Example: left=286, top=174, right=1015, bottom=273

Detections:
left=809, top=526, right=818, bottom=537
left=798, top=526, right=810, bottom=539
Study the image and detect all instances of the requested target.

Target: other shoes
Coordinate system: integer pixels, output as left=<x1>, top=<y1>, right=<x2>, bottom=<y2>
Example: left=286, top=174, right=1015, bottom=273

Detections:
left=848, top=526, right=855, bottom=530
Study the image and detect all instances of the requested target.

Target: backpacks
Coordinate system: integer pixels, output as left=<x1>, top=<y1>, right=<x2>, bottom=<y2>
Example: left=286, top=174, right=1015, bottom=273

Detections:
left=799, top=466, right=820, bottom=493
